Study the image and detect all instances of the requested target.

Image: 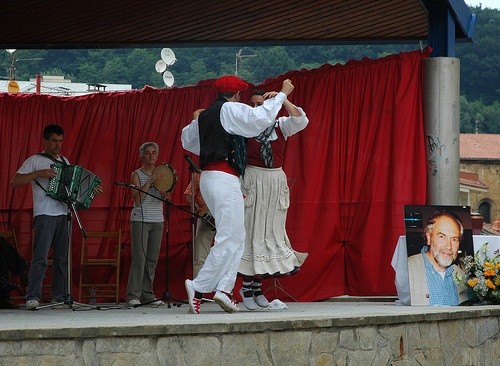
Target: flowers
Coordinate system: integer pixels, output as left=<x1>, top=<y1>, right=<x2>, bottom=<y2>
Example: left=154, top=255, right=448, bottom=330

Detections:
left=455, top=243, right=500, bottom=305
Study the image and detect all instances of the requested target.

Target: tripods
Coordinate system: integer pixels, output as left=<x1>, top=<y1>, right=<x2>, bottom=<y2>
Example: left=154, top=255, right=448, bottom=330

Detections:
left=32, top=185, right=102, bottom=310
left=131, top=187, right=205, bottom=308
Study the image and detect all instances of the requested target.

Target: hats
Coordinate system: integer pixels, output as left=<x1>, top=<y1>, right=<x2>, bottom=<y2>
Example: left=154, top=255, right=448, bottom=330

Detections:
left=213, top=76, right=249, bottom=92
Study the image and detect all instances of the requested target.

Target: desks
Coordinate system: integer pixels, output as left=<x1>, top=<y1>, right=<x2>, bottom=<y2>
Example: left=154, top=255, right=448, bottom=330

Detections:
left=391, top=236, right=500, bottom=306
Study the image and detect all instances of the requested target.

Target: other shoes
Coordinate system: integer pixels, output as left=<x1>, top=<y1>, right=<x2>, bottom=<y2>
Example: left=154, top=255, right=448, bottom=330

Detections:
left=252, top=289, right=269, bottom=307
left=240, top=287, right=257, bottom=310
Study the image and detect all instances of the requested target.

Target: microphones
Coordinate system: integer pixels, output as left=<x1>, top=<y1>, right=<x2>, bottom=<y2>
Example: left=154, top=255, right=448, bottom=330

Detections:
left=115, top=182, right=135, bottom=187
left=185, top=154, right=200, bottom=173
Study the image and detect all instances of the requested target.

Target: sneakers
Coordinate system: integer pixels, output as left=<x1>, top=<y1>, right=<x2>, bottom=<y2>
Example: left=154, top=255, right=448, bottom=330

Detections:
left=213, top=290, right=240, bottom=311
left=27, top=299, right=40, bottom=308
left=128, top=299, right=141, bottom=308
left=185, top=279, right=201, bottom=314
left=144, top=299, right=164, bottom=306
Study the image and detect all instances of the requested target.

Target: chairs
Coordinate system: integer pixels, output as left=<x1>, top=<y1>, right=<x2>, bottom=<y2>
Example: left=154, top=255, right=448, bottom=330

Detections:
left=0, top=228, right=26, bottom=303
left=31, top=226, right=54, bottom=294
left=79, top=229, right=121, bottom=305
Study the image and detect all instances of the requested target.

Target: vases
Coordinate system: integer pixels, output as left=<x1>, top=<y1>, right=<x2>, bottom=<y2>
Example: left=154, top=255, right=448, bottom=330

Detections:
left=480, top=286, right=499, bottom=306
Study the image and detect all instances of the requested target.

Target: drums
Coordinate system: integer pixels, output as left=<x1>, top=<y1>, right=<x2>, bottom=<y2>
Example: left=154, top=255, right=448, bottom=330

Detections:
left=152, top=164, right=176, bottom=193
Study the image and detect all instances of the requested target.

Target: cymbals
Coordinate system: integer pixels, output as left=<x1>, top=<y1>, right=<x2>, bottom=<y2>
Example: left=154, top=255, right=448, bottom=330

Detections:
left=151, top=161, right=179, bottom=194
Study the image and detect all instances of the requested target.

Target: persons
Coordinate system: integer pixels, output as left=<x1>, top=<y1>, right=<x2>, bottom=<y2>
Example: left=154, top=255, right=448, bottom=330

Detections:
left=183, top=170, right=218, bottom=303
left=237, top=90, right=309, bottom=309
left=125, top=142, right=171, bottom=305
left=181, top=75, right=293, bottom=315
left=408, top=210, right=469, bottom=305
left=12, top=124, right=103, bottom=309
